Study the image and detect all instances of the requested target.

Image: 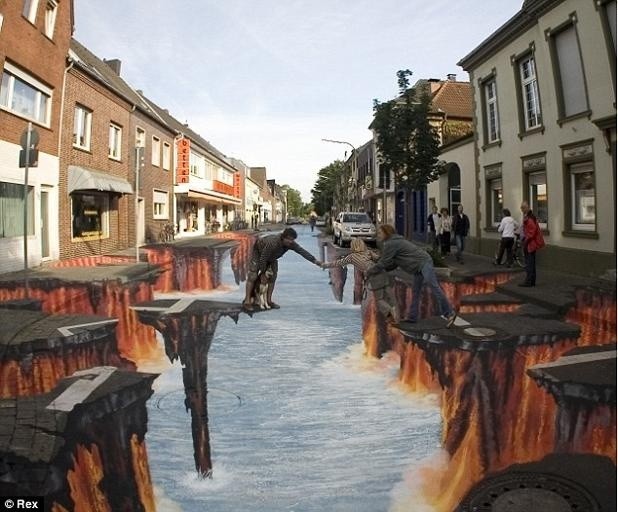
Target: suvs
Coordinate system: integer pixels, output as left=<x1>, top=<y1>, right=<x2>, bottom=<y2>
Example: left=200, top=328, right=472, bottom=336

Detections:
left=329, top=211, right=377, bottom=249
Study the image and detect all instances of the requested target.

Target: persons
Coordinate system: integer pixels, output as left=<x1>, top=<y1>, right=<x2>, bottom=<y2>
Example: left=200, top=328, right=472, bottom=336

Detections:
left=319, top=239, right=401, bottom=326
left=493, top=208, right=518, bottom=268
left=308, top=215, right=315, bottom=231
left=438, top=208, right=452, bottom=260
left=517, top=213, right=529, bottom=271
left=241, top=228, right=319, bottom=311
left=361, top=223, right=456, bottom=329
left=450, top=204, right=470, bottom=265
left=518, top=200, right=544, bottom=287
left=426, top=206, right=441, bottom=253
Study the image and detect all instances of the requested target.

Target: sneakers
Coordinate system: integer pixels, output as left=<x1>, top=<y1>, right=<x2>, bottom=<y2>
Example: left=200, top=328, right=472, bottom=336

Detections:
left=386, top=306, right=414, bottom=323
left=518, top=282, right=532, bottom=287
left=242, top=300, right=253, bottom=312
left=446, top=311, right=456, bottom=328
left=270, top=301, right=280, bottom=310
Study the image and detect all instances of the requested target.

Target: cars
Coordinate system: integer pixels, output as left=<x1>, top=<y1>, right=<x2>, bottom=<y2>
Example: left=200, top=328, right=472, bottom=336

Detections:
left=286, top=215, right=326, bottom=226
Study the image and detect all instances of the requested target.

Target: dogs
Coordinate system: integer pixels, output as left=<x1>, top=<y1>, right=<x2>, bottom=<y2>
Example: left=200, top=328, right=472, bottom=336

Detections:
left=243, top=264, right=274, bottom=309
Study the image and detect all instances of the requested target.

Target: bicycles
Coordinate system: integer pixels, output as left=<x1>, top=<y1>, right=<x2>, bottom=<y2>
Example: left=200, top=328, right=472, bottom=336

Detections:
left=157, top=221, right=177, bottom=243
left=494, top=224, right=528, bottom=270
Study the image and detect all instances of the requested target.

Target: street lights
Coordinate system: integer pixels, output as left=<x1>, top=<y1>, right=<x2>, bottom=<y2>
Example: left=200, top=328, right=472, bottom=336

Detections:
left=320, top=138, right=359, bottom=212
